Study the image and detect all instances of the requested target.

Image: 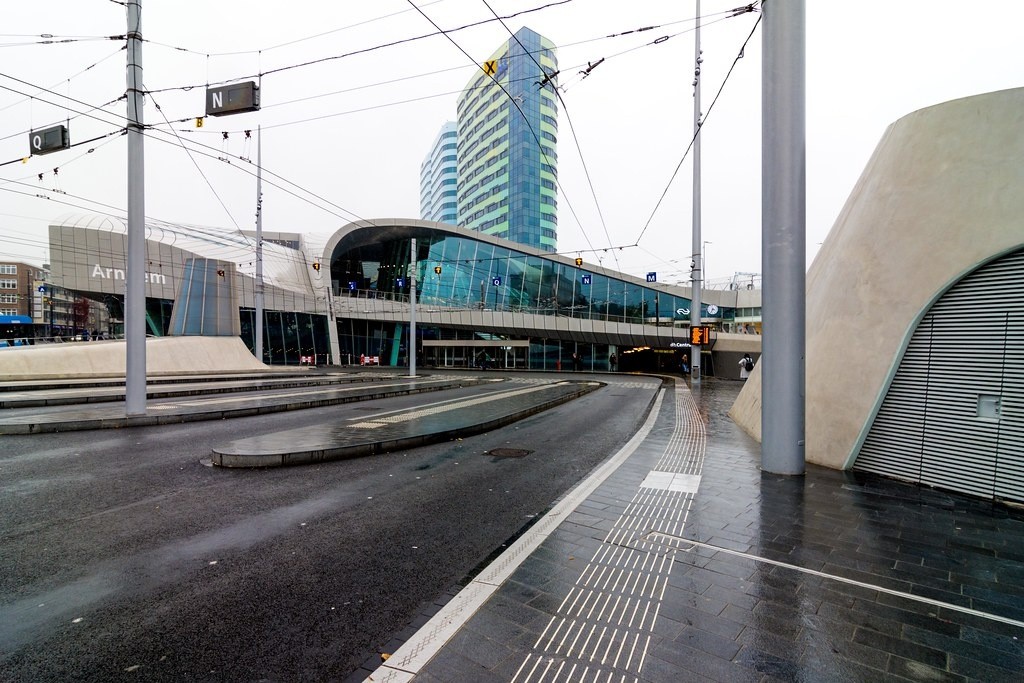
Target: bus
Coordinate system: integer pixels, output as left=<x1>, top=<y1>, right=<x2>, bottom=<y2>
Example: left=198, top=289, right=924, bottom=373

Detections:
left=0, top=311, right=35, bottom=348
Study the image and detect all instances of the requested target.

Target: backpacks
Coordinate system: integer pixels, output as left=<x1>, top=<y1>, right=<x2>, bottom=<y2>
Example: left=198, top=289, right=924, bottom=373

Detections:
left=745, top=357, right=753, bottom=372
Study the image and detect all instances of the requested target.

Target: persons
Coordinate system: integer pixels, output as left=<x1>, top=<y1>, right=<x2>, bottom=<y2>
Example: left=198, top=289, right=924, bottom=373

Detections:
left=679, top=354, right=689, bottom=377
left=572, top=352, right=584, bottom=371
left=658, top=360, right=665, bottom=373
left=417, top=349, right=425, bottom=367
left=478, top=349, right=486, bottom=370
left=738, top=353, right=754, bottom=382
left=608, top=353, right=617, bottom=372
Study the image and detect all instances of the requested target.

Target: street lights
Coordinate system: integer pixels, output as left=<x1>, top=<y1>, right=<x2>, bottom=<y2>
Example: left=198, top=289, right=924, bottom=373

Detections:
left=27, top=269, right=33, bottom=318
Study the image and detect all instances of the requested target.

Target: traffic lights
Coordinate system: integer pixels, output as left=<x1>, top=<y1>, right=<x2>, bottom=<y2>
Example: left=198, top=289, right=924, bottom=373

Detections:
left=690, top=325, right=709, bottom=346
